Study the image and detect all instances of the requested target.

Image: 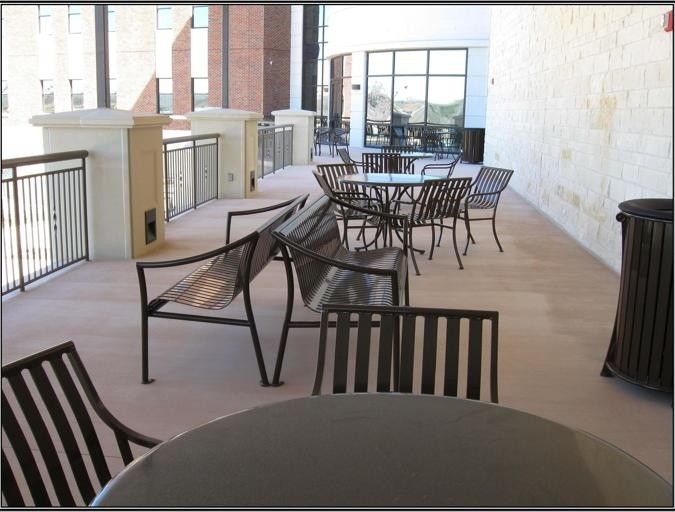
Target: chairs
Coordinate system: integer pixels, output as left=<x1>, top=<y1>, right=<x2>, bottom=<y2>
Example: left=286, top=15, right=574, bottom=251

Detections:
left=270, top=191, right=414, bottom=385
left=311, top=144, right=516, bottom=277
left=1, top=340, right=166, bottom=510
left=311, top=304, right=499, bottom=406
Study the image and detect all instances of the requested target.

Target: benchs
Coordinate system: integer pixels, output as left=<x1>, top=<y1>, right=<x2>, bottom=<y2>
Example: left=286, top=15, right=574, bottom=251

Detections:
left=135, top=191, right=309, bottom=386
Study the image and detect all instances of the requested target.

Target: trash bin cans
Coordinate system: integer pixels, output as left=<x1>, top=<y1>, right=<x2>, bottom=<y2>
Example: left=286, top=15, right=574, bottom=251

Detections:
left=460, top=128, right=484, bottom=163
left=600, top=199, right=674, bottom=393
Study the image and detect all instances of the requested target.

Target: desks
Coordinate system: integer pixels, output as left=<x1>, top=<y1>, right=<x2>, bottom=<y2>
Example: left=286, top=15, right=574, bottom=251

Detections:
left=89, top=392, right=674, bottom=510
